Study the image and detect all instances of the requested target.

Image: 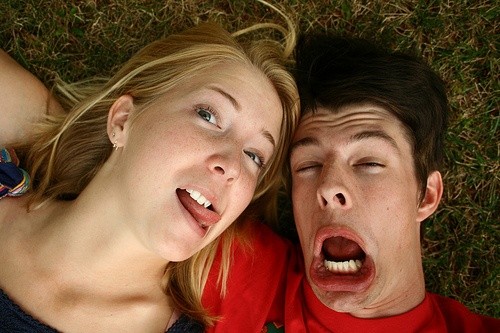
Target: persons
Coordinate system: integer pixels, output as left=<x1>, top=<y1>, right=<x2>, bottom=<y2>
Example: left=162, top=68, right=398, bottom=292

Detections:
left=0, top=2, right=304, bottom=333
left=0, top=42, right=500, bottom=333
